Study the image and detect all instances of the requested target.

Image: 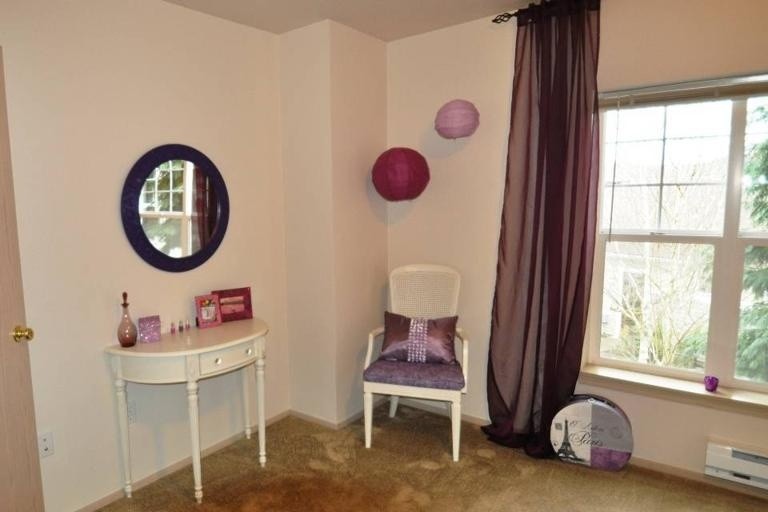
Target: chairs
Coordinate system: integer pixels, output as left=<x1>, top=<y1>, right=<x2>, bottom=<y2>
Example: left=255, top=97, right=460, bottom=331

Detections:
left=363, top=263, right=469, bottom=461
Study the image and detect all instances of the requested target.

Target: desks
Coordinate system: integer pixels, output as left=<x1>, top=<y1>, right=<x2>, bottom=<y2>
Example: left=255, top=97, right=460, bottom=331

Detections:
left=105, top=312, right=267, bottom=499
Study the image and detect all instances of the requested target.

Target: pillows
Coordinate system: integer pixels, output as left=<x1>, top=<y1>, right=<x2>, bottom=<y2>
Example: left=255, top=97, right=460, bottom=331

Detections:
left=380, top=310, right=459, bottom=366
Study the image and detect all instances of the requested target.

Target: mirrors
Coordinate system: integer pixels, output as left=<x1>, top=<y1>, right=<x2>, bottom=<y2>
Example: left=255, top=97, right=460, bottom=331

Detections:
left=120, top=143, right=230, bottom=273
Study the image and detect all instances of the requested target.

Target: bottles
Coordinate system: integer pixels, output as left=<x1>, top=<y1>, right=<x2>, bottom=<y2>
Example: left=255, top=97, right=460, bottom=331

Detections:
left=117, top=292, right=138, bottom=347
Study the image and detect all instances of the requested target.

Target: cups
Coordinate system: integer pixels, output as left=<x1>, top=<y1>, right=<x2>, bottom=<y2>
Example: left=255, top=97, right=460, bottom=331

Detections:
left=705, top=376, right=718, bottom=393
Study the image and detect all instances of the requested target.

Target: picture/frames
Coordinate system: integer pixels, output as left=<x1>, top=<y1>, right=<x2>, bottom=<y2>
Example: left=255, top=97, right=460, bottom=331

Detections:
left=195, top=294, right=221, bottom=328
left=212, top=287, right=253, bottom=322
